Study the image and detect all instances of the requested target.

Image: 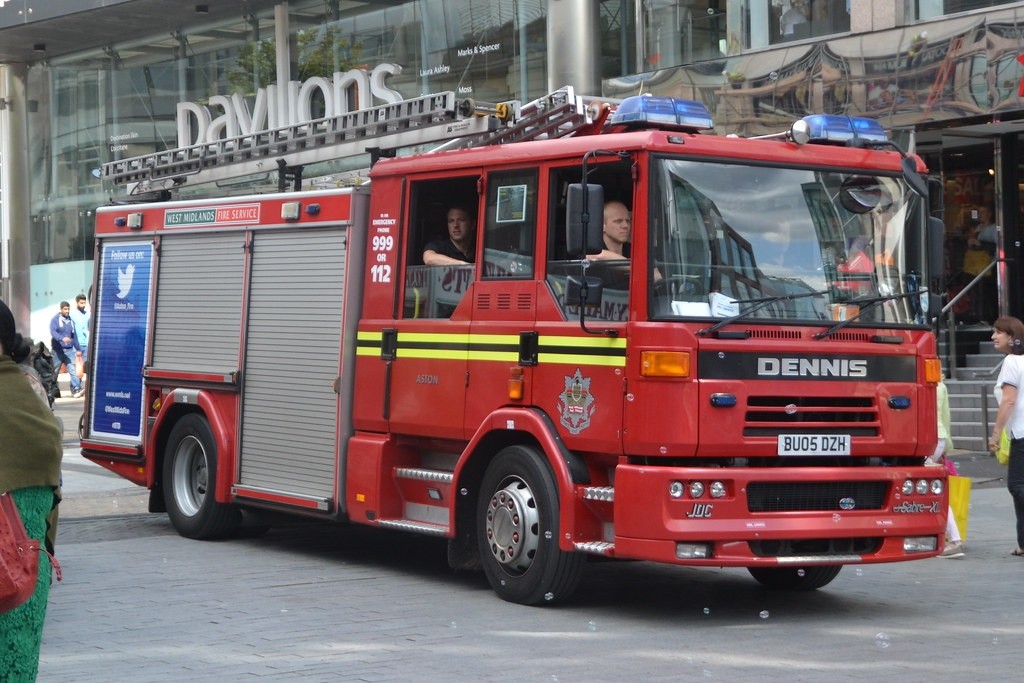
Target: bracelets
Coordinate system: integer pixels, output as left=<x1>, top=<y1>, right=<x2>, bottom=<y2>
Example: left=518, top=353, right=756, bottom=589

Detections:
left=992, top=431, right=1000, bottom=436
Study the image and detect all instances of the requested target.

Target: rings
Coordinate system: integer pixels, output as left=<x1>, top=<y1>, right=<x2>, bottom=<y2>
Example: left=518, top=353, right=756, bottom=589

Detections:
left=993, top=444, right=997, bottom=449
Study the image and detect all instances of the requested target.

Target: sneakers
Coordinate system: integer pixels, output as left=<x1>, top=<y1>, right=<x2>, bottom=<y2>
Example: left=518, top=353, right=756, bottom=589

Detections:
left=935, top=542, right=967, bottom=561
left=73, top=388, right=85, bottom=398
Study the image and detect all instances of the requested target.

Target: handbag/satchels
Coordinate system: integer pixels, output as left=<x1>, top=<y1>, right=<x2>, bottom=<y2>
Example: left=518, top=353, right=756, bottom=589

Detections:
left=0, top=492, right=63, bottom=617
left=993, top=423, right=1012, bottom=465
left=74, top=353, right=84, bottom=380
left=947, top=475, right=971, bottom=541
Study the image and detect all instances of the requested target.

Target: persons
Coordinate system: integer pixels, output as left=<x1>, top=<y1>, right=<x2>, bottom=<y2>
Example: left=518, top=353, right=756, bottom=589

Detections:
left=586, top=202, right=660, bottom=282
left=70, top=294, right=90, bottom=394
left=22, top=337, right=61, bottom=408
left=50, top=301, right=85, bottom=397
left=755, top=214, right=790, bottom=266
left=964, top=204, right=998, bottom=326
left=987, top=316, right=1024, bottom=554
left=924, top=375, right=965, bottom=558
left=0, top=299, right=63, bottom=683
left=423, top=204, right=475, bottom=265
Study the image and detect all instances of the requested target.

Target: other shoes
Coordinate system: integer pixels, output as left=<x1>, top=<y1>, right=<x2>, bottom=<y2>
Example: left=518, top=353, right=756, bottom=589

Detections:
left=1009, top=546, right=1024, bottom=556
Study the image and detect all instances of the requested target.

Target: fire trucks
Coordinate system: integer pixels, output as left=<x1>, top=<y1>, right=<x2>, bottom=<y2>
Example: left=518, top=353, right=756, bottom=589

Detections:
left=70, top=84, right=1002, bottom=607
